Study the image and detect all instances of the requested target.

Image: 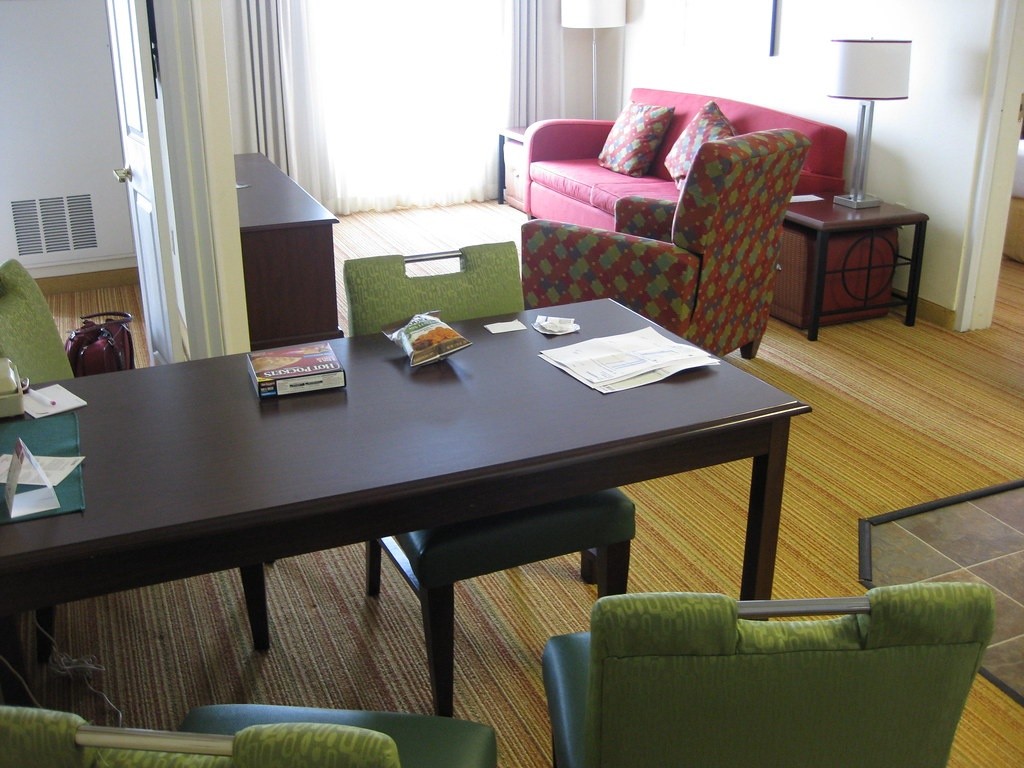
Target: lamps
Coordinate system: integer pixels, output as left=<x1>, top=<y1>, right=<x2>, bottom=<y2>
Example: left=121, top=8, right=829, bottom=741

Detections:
left=828, top=39, right=912, bottom=209
left=561, top=0, right=626, bottom=120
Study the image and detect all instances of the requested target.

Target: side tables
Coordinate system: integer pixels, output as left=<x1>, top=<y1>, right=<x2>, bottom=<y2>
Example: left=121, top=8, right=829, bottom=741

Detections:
left=497, top=127, right=528, bottom=214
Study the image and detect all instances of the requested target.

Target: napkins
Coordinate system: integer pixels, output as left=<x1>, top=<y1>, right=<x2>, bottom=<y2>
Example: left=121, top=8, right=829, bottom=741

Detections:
left=0, top=411, right=86, bottom=525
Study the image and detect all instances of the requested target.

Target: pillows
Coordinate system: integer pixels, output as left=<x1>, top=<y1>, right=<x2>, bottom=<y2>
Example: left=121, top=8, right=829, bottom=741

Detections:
left=598, top=99, right=674, bottom=175
left=664, top=100, right=736, bottom=192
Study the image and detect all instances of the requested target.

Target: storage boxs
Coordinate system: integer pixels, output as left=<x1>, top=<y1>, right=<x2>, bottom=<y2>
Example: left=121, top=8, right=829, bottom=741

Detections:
left=769, top=225, right=899, bottom=328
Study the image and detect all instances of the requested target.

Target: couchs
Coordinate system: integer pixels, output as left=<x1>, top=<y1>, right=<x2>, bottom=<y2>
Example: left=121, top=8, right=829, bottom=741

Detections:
left=523, top=87, right=847, bottom=233
left=521, top=127, right=811, bottom=360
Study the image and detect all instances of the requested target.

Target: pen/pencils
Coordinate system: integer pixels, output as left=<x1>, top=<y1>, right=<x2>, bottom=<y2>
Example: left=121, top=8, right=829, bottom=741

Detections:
left=21, top=382, right=57, bottom=406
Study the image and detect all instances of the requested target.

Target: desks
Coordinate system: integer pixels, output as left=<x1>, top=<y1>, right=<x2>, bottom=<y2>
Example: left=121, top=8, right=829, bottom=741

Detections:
left=784, top=191, right=930, bottom=342
left=0, top=298, right=811, bottom=619
left=235, top=152, right=342, bottom=352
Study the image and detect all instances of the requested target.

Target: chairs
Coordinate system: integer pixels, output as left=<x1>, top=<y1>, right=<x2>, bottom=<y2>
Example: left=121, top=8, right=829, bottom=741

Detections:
left=0, top=257, right=270, bottom=663
left=343, top=242, right=636, bottom=718
left=542, top=581, right=996, bottom=768
left=0, top=701, right=498, bottom=768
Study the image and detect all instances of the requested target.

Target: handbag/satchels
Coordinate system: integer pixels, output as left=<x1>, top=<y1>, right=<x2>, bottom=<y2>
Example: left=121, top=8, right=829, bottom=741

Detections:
left=65, top=311, right=135, bottom=379
